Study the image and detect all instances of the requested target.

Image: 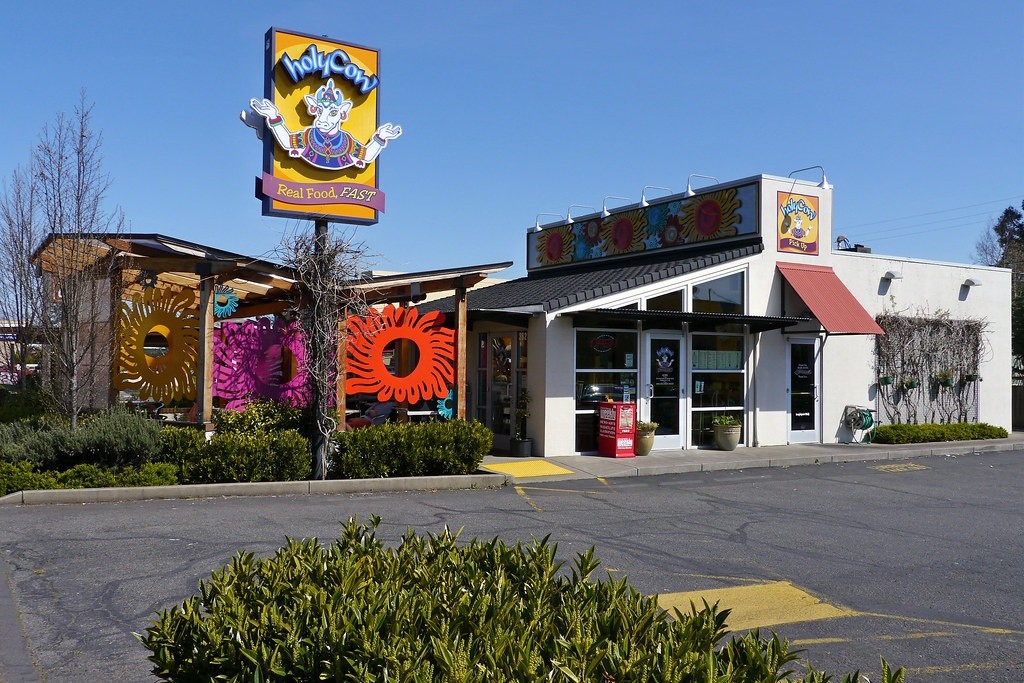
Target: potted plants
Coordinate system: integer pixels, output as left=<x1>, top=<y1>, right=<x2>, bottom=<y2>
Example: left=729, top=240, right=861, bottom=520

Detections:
left=879, top=368, right=892, bottom=385
left=966, top=370, right=977, bottom=381
left=712, top=415, right=742, bottom=451
left=636, top=420, right=659, bottom=455
left=937, top=369, right=954, bottom=387
left=509, top=388, right=534, bottom=457
left=904, top=375, right=917, bottom=389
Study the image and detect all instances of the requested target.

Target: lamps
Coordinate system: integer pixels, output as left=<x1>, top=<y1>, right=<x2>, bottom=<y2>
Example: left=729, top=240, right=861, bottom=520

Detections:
left=885, top=271, right=903, bottom=279
left=638, top=186, right=673, bottom=208
left=964, top=279, right=982, bottom=286
left=533, top=213, right=564, bottom=232
left=600, top=196, right=633, bottom=218
left=565, top=205, right=596, bottom=224
left=788, top=166, right=829, bottom=190
left=684, top=174, right=719, bottom=198
